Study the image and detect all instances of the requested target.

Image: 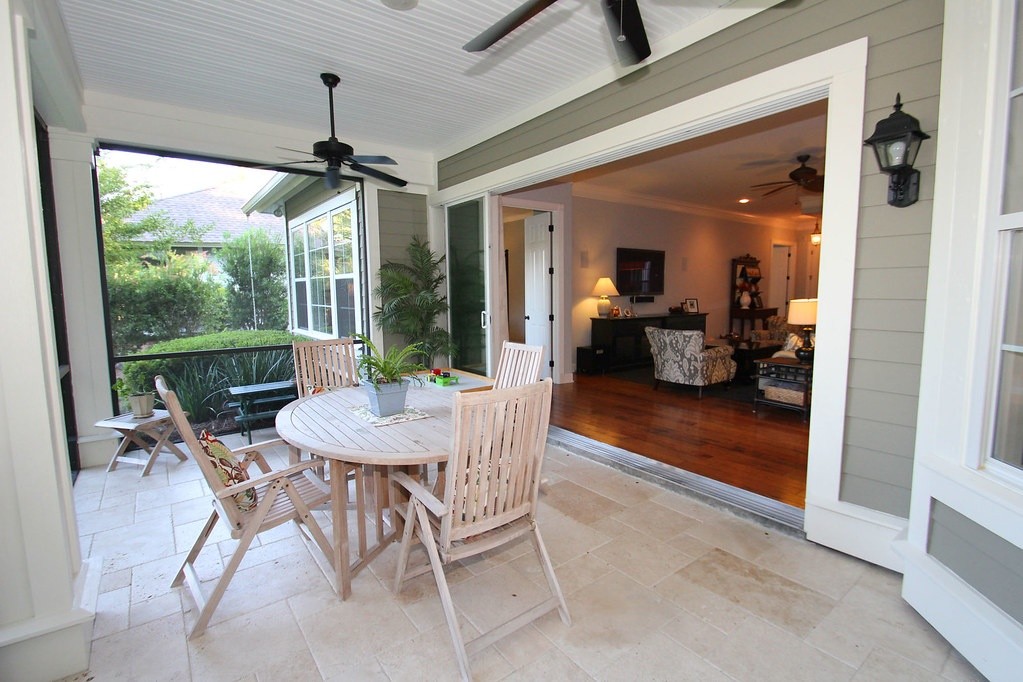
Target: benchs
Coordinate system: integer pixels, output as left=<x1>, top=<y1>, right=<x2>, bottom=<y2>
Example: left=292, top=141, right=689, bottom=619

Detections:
left=228, top=394, right=298, bottom=445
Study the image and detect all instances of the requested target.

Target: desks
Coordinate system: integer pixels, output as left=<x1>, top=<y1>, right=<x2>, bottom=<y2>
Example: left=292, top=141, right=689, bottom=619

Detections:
left=94, top=408, right=190, bottom=478
left=276, top=372, right=493, bottom=601
left=730, top=306, right=779, bottom=340
left=228, top=377, right=304, bottom=444
left=702, top=335, right=786, bottom=401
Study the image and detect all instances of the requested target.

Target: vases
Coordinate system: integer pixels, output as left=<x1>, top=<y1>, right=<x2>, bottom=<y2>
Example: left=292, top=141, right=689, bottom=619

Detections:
left=740, top=291, right=753, bottom=310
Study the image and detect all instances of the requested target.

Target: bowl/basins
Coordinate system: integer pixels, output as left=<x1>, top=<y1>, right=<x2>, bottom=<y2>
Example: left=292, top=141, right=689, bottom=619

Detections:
left=668, top=306, right=683, bottom=314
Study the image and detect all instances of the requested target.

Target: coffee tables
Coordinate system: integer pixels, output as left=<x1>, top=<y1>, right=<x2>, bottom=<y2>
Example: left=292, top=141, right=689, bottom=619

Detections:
left=750, top=360, right=813, bottom=424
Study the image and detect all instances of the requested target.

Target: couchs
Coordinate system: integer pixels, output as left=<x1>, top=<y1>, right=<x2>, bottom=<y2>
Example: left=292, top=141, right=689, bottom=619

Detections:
left=752, top=314, right=814, bottom=358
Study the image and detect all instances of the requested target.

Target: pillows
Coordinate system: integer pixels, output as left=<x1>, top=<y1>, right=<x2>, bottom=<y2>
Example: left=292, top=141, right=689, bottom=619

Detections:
left=199, top=430, right=261, bottom=513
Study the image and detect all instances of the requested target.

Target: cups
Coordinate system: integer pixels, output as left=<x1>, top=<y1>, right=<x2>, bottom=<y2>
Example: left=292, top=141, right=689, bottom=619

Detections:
left=611, top=309, right=620, bottom=318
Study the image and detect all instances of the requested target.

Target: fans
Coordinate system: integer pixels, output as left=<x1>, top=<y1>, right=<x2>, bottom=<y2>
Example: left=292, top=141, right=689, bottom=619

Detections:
left=250, top=72, right=407, bottom=191
left=461, top=0, right=652, bottom=66
left=750, top=154, right=825, bottom=200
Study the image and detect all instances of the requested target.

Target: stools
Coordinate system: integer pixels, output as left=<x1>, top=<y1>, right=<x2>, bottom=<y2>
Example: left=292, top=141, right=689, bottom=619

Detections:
left=577, top=345, right=606, bottom=375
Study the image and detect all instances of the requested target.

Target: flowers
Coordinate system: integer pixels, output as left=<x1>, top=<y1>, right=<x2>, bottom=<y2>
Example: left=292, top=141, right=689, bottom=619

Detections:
left=736, top=278, right=752, bottom=293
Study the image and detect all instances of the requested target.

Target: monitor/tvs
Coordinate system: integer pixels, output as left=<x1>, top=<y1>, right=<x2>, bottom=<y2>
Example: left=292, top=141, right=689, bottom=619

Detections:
left=616, top=248, right=664, bottom=296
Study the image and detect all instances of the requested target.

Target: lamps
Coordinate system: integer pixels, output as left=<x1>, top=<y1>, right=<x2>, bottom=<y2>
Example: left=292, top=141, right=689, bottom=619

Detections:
left=591, top=277, right=620, bottom=318
left=787, top=297, right=819, bottom=365
left=864, top=91, right=930, bottom=206
left=810, top=217, right=822, bottom=246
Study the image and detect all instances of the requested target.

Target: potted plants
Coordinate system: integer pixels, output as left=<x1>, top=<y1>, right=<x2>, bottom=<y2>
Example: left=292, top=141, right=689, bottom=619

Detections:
left=350, top=331, right=432, bottom=418
left=110, top=372, right=156, bottom=418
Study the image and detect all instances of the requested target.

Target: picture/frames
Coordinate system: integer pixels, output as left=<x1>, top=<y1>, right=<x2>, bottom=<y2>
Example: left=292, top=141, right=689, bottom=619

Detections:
left=681, top=297, right=699, bottom=313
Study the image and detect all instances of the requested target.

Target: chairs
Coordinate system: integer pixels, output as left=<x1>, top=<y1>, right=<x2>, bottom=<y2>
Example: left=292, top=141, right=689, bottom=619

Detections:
left=646, top=327, right=736, bottom=399
left=291, top=332, right=573, bottom=681
left=153, top=374, right=342, bottom=641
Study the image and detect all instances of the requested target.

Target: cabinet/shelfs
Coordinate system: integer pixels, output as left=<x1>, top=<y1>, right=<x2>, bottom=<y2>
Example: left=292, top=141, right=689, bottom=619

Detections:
left=590, top=313, right=710, bottom=377
